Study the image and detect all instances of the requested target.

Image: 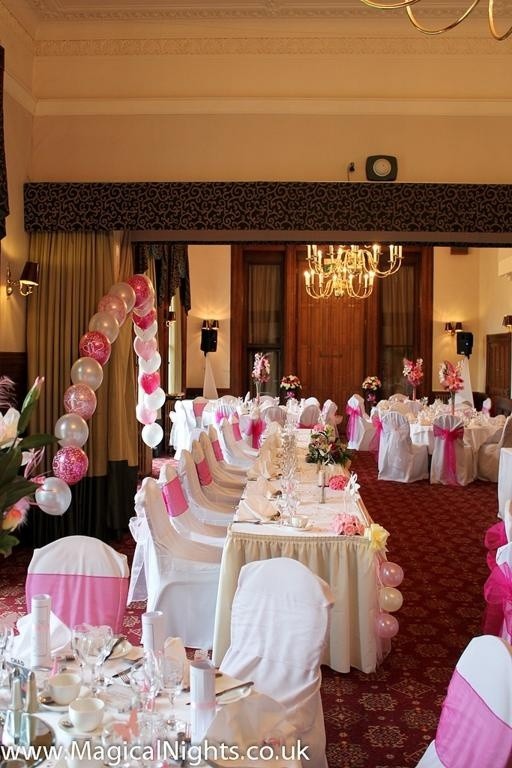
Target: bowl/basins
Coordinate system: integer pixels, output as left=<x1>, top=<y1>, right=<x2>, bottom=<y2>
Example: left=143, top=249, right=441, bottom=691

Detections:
left=0, top=709, right=56, bottom=768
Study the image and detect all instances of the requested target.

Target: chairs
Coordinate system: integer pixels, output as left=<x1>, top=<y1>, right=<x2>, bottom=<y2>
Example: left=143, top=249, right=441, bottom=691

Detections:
left=22, top=382, right=512, bottom=676
left=217, top=557, right=337, bottom=768
left=412, top=633, right=512, bottom=768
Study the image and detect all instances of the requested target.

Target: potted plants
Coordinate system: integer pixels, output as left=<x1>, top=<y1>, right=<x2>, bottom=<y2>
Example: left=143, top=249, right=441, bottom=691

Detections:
left=361, top=374, right=382, bottom=392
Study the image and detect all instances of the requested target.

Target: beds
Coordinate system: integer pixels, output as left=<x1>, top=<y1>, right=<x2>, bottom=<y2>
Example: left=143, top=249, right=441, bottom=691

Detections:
left=2, top=633, right=303, bottom=768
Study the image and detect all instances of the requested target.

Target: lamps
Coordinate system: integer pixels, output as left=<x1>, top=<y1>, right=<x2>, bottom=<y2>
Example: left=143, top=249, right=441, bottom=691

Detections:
left=501, top=312, right=512, bottom=333
left=5, top=259, right=39, bottom=302
left=200, top=316, right=220, bottom=360
left=303, top=243, right=405, bottom=302
left=444, top=319, right=474, bottom=362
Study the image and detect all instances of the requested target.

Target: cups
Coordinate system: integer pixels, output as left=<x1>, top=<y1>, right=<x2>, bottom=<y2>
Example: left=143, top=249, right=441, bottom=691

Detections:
left=67, top=697, right=105, bottom=730
left=47, top=672, right=82, bottom=706
left=292, top=515, right=309, bottom=527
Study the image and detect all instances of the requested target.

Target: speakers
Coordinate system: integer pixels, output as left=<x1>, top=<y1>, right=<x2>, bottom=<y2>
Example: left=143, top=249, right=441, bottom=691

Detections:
left=457, top=332, right=473, bottom=354
left=200, top=329, right=217, bottom=352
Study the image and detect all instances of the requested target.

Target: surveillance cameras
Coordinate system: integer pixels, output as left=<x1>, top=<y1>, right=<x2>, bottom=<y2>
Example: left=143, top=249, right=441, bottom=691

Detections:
left=348, top=162, right=356, bottom=171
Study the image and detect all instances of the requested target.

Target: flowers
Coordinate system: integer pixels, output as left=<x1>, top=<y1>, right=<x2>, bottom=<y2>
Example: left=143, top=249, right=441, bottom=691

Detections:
left=252, top=348, right=271, bottom=383
left=402, top=354, right=426, bottom=387
left=280, top=370, right=303, bottom=392
left=438, top=356, right=468, bottom=393
left=0, top=374, right=65, bottom=557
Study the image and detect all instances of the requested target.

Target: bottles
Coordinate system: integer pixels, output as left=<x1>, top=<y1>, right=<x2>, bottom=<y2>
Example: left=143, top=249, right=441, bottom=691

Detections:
left=308, top=429, right=314, bottom=447
left=8, top=665, right=24, bottom=710
left=319, top=483, right=326, bottom=504
left=24, top=669, right=40, bottom=714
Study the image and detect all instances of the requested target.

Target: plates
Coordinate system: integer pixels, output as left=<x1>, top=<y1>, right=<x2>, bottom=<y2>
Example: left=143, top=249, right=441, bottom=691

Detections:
left=59, top=713, right=114, bottom=735
left=109, top=638, right=133, bottom=659
left=37, top=688, right=97, bottom=714
left=293, top=525, right=313, bottom=530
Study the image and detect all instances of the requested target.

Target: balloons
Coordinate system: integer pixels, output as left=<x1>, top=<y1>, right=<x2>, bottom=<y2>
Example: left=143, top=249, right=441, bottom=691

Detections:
left=378, top=588, right=402, bottom=612
left=375, top=616, right=400, bottom=637
left=380, top=561, right=405, bottom=586
left=38, top=273, right=168, bottom=516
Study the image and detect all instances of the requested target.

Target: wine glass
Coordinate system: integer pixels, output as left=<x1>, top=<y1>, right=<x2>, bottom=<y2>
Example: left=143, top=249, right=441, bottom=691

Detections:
left=71, top=622, right=113, bottom=695
left=414, top=403, right=484, bottom=427
left=103, top=649, right=256, bottom=767
left=274, top=418, right=298, bottom=528
left=234, top=394, right=281, bottom=416
